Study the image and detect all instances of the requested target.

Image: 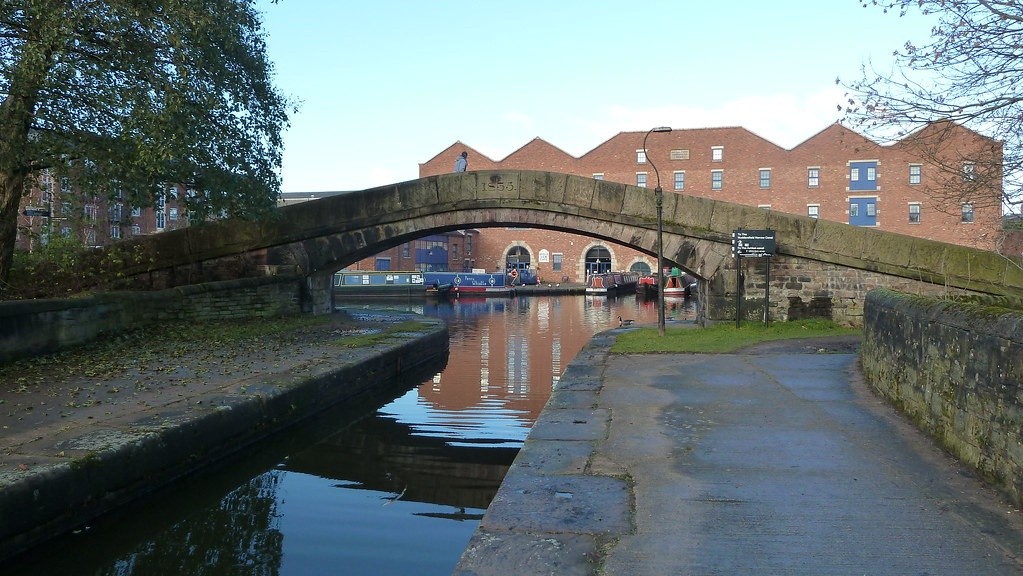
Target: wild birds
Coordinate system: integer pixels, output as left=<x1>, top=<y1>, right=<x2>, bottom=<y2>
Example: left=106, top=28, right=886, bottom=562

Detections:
left=618, top=316, right=634, bottom=325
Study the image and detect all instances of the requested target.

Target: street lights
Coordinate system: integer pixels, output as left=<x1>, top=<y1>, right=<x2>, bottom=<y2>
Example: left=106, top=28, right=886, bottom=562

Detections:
left=643, top=126, right=672, bottom=328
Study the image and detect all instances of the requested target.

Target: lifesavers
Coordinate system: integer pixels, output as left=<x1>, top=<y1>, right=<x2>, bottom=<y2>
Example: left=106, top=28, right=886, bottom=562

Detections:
left=511, top=268, right=519, bottom=278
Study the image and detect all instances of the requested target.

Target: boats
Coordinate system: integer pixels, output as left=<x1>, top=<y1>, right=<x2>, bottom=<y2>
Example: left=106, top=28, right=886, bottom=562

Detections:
left=422, top=270, right=517, bottom=293
left=334, top=271, right=452, bottom=297
left=636, top=272, right=658, bottom=293
left=664, top=267, right=695, bottom=294
left=585, top=270, right=639, bottom=296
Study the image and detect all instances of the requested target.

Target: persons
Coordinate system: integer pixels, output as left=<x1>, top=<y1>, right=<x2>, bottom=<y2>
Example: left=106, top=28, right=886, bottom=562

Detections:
left=453, top=151, right=468, bottom=172
